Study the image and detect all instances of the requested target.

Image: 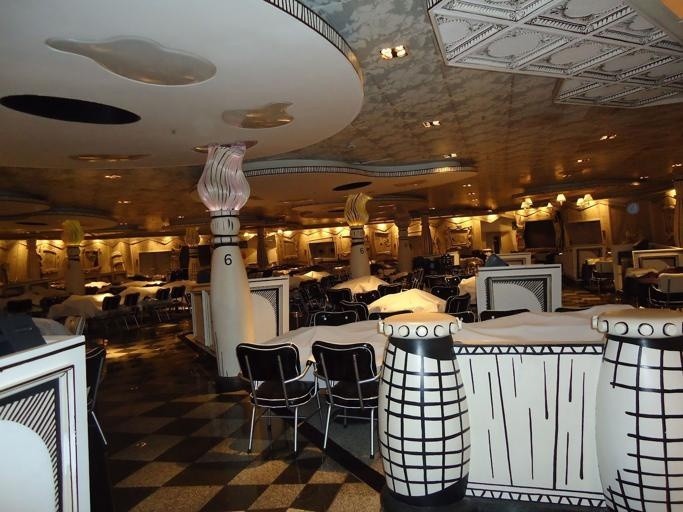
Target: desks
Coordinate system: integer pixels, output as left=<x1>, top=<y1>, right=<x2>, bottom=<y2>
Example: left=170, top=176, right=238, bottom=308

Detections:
left=254, top=318, right=387, bottom=387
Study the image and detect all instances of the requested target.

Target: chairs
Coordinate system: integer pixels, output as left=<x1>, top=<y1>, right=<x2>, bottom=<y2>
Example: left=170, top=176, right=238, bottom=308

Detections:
left=311, top=340, right=381, bottom=457
left=235, top=341, right=321, bottom=455
left=2, top=245, right=202, bottom=457
left=239, top=247, right=683, bottom=328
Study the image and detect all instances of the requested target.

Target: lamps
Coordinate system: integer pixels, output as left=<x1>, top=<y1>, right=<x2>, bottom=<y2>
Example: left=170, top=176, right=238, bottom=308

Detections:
left=517, top=193, right=595, bottom=220
